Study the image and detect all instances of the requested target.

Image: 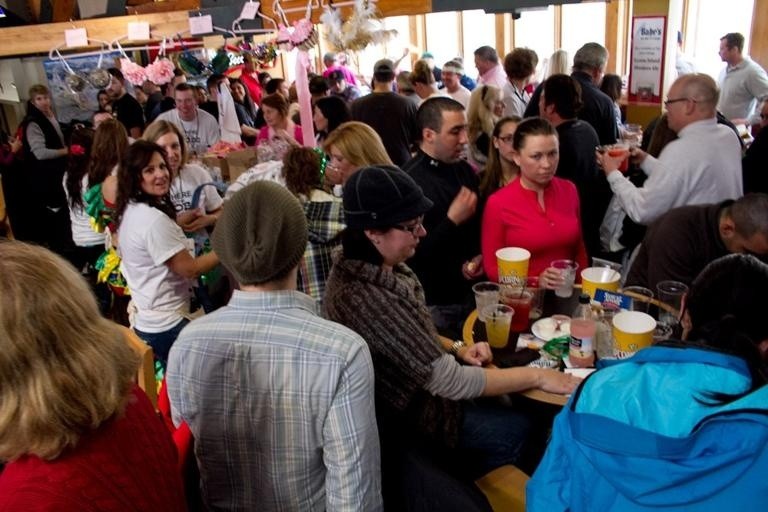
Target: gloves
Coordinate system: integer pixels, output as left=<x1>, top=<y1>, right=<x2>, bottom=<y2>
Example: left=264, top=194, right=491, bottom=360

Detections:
left=69, top=144, right=87, bottom=158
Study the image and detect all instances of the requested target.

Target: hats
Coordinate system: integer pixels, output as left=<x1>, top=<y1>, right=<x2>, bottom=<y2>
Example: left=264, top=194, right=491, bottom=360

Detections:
left=374, top=58, right=393, bottom=73
left=211, top=180, right=310, bottom=282
left=328, top=70, right=344, bottom=82
left=342, top=165, right=435, bottom=226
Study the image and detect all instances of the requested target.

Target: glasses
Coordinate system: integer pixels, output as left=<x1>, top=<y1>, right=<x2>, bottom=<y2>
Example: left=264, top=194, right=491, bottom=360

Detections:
left=394, top=216, right=427, bottom=238
left=495, top=135, right=514, bottom=146
left=664, top=98, right=693, bottom=108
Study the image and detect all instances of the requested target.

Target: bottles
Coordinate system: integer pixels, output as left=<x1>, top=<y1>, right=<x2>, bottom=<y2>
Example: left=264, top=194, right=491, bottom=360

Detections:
left=568, top=293, right=597, bottom=368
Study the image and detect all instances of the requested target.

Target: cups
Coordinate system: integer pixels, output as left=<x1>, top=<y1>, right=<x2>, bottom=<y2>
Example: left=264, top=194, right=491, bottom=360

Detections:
left=590, top=257, right=623, bottom=284
left=622, top=286, right=655, bottom=315
left=550, top=258, right=580, bottom=299
left=656, top=279, right=689, bottom=326
left=473, top=276, right=548, bottom=349
left=594, top=122, right=643, bottom=173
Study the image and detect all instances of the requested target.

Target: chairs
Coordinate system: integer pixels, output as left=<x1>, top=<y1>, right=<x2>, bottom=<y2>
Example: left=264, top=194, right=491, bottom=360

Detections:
left=114, top=321, right=156, bottom=406
left=473, top=463, right=532, bottom=512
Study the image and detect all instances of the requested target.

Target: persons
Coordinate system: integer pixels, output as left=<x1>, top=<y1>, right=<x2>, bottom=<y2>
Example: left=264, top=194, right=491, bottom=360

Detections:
left=0, top=31, right=768, bottom=512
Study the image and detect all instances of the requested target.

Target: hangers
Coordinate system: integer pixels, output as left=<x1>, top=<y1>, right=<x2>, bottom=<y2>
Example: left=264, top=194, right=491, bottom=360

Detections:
left=49, top=19, right=111, bottom=59
left=170, top=6, right=238, bottom=46
left=112, top=8, right=174, bottom=51
left=232, top=0, right=279, bottom=33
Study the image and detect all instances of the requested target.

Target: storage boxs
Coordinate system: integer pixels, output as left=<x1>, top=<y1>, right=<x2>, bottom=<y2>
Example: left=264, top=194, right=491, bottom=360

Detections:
left=198, top=146, right=257, bottom=185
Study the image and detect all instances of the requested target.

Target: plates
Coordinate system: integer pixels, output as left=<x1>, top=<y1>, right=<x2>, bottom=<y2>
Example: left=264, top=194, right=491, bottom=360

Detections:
left=531, top=315, right=573, bottom=343
